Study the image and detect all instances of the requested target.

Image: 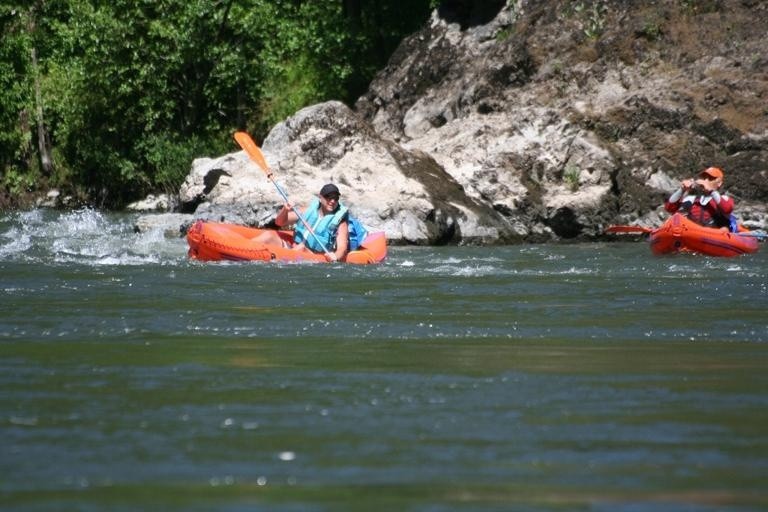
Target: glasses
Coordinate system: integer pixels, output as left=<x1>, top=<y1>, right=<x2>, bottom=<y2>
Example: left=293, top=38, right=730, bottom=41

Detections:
left=324, top=194, right=339, bottom=200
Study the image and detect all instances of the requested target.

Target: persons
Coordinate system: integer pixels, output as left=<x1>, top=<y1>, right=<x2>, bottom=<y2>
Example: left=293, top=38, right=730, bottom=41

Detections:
left=275, top=183, right=352, bottom=263
left=662, top=165, right=734, bottom=230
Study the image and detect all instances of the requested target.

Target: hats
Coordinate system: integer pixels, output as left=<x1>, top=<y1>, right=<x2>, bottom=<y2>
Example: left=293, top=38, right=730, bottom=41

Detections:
left=320, top=184, right=340, bottom=196
left=697, top=166, right=723, bottom=177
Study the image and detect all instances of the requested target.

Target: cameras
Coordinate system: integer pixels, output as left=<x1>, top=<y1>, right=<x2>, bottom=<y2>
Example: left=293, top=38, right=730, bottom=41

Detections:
left=690, top=182, right=702, bottom=191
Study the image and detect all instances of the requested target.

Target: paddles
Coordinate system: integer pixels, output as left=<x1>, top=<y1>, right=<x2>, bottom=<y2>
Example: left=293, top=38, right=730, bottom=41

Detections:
left=234, top=131, right=330, bottom=255
left=603, top=225, right=768, bottom=238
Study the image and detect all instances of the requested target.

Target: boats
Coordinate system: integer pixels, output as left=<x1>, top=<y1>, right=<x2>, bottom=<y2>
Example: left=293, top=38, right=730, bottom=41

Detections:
left=647, top=212, right=758, bottom=259
left=182, top=217, right=390, bottom=266
